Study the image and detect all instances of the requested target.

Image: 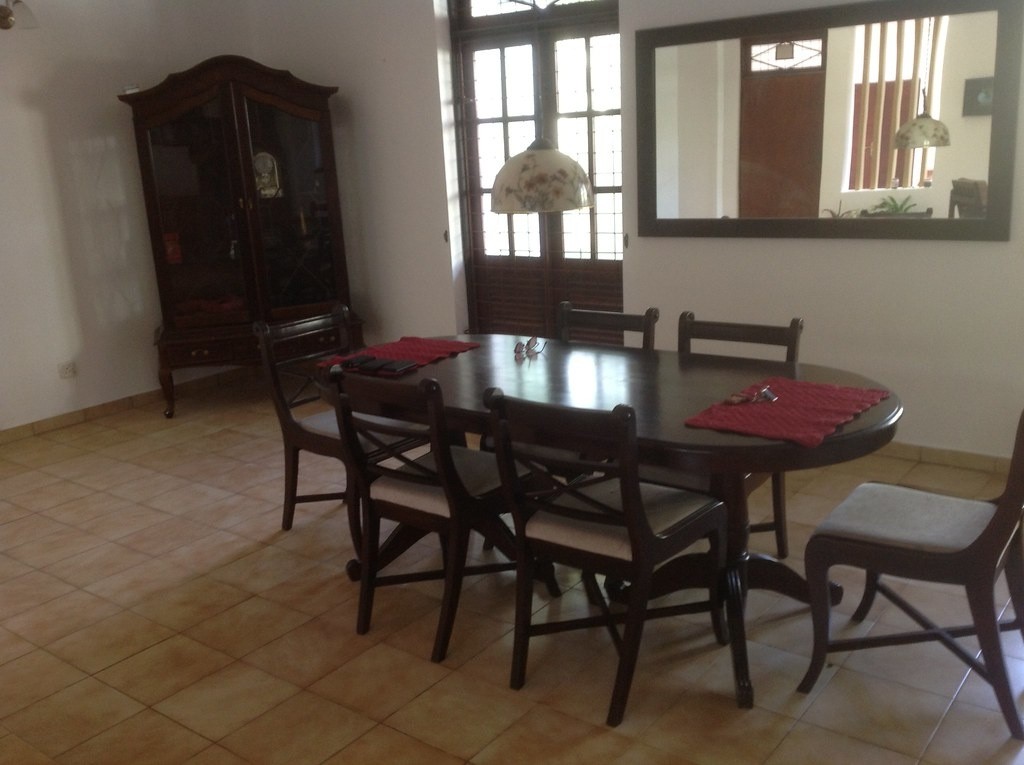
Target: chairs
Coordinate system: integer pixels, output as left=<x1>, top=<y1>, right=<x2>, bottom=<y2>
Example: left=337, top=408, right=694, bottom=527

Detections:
left=329, top=364, right=559, bottom=664
left=677, top=309, right=804, bottom=558
left=795, top=408, right=1024, bottom=745
left=481, top=385, right=731, bottom=726
left=557, top=301, right=660, bottom=347
left=251, top=304, right=430, bottom=558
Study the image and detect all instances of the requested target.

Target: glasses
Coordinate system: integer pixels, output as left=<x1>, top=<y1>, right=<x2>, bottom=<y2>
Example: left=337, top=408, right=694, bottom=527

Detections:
left=515, top=337, right=547, bottom=352
left=514, top=351, right=545, bottom=361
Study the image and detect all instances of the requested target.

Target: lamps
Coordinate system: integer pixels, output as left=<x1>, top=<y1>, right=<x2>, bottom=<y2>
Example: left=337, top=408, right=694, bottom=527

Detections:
left=492, top=1, right=593, bottom=214
left=891, top=16, right=951, bottom=149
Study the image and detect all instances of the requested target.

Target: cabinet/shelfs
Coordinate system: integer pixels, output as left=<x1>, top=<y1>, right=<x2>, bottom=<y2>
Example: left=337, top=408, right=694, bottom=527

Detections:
left=117, top=54, right=366, bottom=419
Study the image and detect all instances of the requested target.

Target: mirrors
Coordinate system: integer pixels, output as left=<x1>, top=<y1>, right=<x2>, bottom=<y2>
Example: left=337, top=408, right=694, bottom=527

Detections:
left=635, top=0, right=1024, bottom=243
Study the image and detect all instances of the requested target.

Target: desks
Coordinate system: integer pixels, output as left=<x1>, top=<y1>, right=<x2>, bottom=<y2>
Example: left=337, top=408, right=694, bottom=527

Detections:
left=325, top=332, right=903, bottom=709
